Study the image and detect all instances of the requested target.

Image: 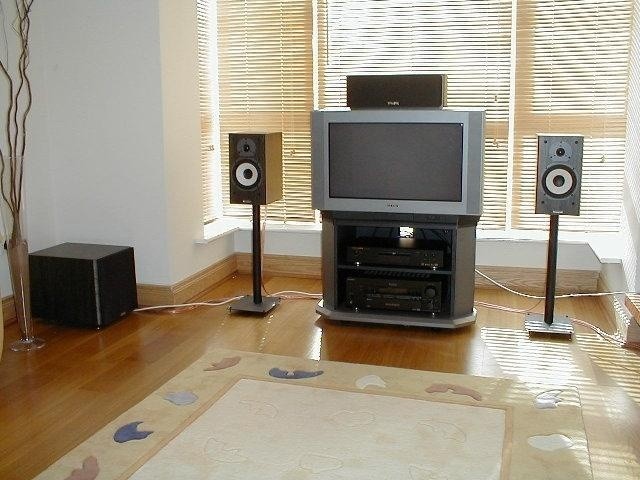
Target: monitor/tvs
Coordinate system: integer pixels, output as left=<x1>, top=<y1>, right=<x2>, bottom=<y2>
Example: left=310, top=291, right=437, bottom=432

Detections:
left=311, top=110, right=486, bottom=225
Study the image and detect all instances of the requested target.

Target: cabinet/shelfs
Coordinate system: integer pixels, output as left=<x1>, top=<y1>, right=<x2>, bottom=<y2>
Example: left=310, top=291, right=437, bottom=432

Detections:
left=316, top=212, right=481, bottom=331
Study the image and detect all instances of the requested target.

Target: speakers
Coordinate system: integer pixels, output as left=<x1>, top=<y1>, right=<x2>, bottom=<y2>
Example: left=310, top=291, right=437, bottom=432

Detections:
left=28, top=242, right=139, bottom=332
left=534, top=133, right=584, bottom=216
left=346, top=74, right=448, bottom=109
left=228, top=131, right=283, bottom=205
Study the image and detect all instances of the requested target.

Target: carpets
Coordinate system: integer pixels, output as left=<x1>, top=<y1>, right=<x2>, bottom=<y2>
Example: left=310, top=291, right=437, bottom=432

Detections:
left=17, top=347, right=592, bottom=480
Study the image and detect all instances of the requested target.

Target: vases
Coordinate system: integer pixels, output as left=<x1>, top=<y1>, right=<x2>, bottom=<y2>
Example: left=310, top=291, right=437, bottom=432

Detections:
left=1, top=153, right=46, bottom=354
